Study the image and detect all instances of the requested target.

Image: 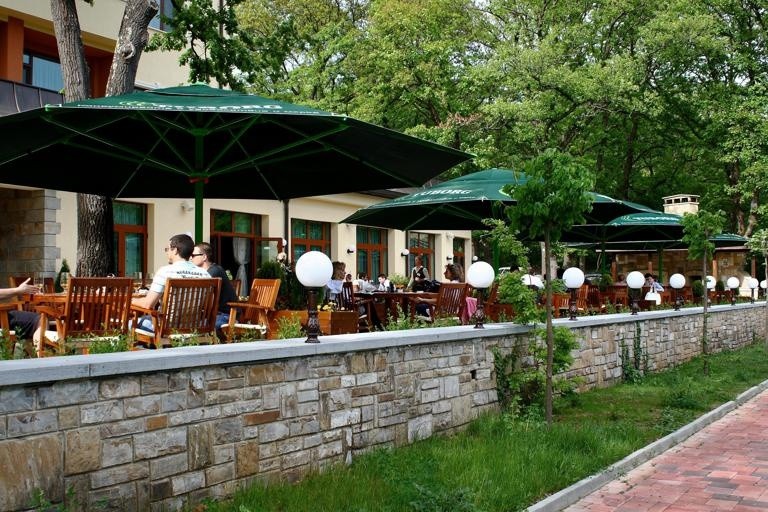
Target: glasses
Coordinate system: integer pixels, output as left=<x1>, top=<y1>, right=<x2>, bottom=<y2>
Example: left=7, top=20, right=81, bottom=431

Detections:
left=191, top=254, right=203, bottom=257
left=164, top=248, right=174, bottom=252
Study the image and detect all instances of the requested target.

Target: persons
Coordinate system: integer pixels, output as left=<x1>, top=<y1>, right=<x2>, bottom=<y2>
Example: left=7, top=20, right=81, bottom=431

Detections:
left=119, top=234, right=215, bottom=348
left=191, top=241, right=240, bottom=344
left=1, top=278, right=56, bottom=359
left=406, top=255, right=482, bottom=320
left=511, top=266, right=665, bottom=304
left=329, top=263, right=396, bottom=331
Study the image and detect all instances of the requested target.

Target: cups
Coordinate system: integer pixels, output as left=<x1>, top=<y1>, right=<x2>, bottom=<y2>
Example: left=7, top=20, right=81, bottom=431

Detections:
left=144, top=271, right=154, bottom=289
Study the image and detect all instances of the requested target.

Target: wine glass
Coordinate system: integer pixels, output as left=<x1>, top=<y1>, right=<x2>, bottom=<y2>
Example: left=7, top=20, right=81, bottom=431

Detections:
left=130, top=271, right=143, bottom=296
left=59, top=272, right=73, bottom=295
left=33, top=271, right=45, bottom=295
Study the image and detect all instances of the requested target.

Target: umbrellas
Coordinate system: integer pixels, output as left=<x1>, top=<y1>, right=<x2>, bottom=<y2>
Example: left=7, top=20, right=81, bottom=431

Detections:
left=670, top=233, right=751, bottom=250
left=567, top=210, right=698, bottom=271
left=339, top=167, right=661, bottom=274
left=0, top=85, right=477, bottom=246
